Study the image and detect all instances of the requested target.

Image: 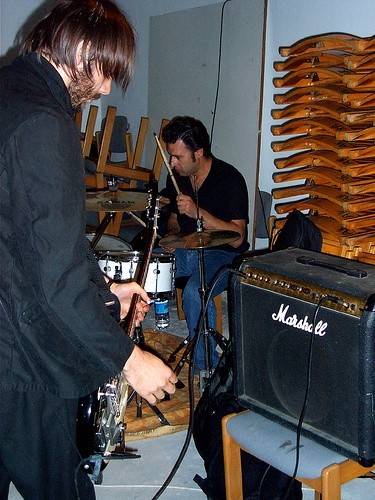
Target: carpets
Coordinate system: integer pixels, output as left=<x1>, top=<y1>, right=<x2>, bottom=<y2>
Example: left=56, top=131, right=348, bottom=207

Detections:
left=122, top=325, right=202, bottom=442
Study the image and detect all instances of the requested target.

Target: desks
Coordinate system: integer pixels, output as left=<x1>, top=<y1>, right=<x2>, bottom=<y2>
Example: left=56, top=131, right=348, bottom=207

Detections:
left=175, top=275, right=224, bottom=336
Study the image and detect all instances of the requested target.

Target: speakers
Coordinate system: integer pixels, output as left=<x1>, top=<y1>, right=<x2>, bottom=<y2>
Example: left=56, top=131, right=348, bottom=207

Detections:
left=233, top=245, right=375, bottom=468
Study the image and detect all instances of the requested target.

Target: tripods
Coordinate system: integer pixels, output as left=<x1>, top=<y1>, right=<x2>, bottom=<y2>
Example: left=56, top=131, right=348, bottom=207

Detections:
left=168, top=249, right=228, bottom=378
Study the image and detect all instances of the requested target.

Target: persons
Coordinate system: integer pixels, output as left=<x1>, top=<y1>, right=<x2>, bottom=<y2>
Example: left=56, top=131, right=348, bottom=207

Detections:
left=152, top=115, right=249, bottom=378
left=0, top=0, right=178, bottom=500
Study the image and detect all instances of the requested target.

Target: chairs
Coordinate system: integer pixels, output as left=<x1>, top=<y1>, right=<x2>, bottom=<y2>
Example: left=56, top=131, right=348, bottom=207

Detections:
left=268, top=30, right=375, bottom=267
left=218, top=409, right=375, bottom=500
left=76, top=103, right=170, bottom=237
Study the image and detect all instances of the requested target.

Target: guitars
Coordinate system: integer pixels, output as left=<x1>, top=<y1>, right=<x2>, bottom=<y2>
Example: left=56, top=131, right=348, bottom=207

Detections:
left=78, top=178, right=163, bottom=461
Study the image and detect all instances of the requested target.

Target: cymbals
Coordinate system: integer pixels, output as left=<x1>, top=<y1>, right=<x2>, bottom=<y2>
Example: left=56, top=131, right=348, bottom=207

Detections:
left=83, top=189, right=171, bottom=212
left=158, top=229, right=242, bottom=250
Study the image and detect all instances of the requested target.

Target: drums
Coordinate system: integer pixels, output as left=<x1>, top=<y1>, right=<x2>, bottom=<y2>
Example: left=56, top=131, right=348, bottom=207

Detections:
left=97, top=250, right=177, bottom=294
left=83, top=231, right=132, bottom=250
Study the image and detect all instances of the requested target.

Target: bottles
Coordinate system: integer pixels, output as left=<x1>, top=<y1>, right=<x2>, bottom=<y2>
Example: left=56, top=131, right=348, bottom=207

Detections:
left=154, top=293, right=171, bottom=330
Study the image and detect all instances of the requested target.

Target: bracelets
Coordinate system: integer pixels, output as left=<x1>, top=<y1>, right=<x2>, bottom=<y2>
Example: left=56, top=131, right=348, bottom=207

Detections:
left=108, top=279, right=113, bottom=286
